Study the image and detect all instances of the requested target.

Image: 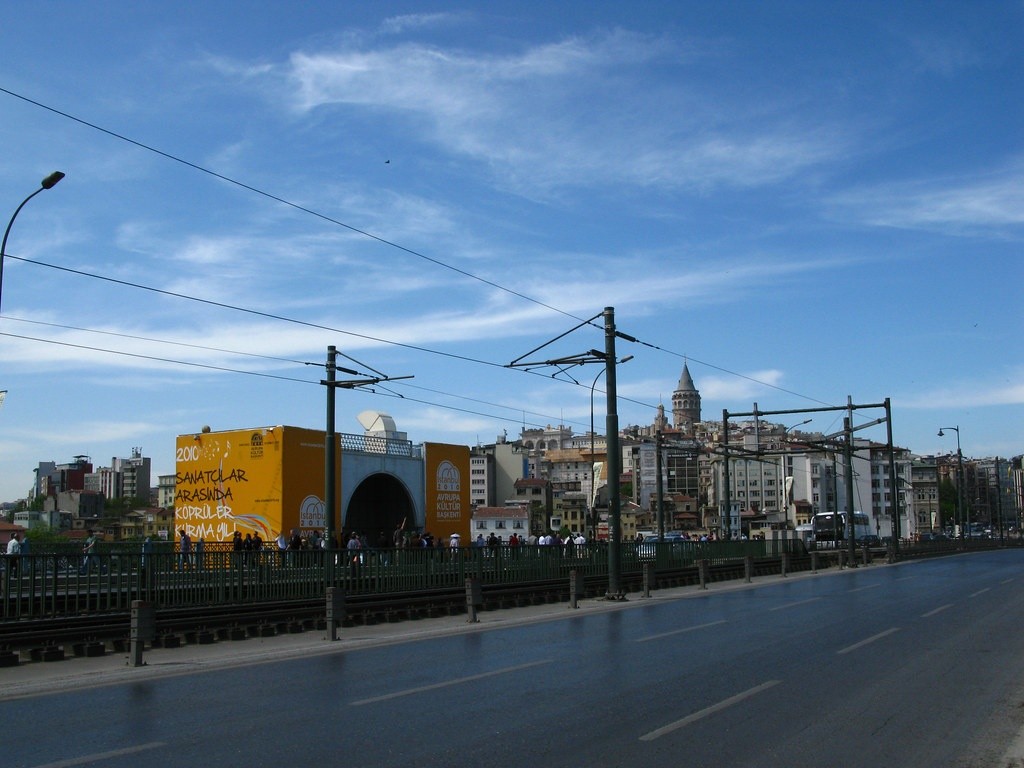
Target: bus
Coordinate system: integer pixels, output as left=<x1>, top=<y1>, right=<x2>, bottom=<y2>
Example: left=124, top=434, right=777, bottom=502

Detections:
left=810, top=511, right=870, bottom=550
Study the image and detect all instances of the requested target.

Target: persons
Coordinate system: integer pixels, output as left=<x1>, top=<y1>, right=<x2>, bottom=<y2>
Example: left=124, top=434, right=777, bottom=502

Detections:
left=488, top=533, right=520, bottom=559
left=6, top=533, right=24, bottom=579
left=679, top=531, right=765, bottom=542
left=347, top=531, right=364, bottom=566
left=636, top=533, right=643, bottom=548
left=393, top=517, right=445, bottom=565
left=476, top=534, right=484, bottom=555
left=449, top=533, right=461, bottom=563
left=271, top=528, right=324, bottom=568
left=178, top=530, right=194, bottom=572
left=233, top=531, right=264, bottom=570
left=528, top=532, right=606, bottom=559
left=79, top=530, right=109, bottom=576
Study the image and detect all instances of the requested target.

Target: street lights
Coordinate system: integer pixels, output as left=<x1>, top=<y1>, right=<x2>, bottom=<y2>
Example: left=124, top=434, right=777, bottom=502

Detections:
left=938, top=425, right=964, bottom=540
left=783, top=418, right=813, bottom=529
left=592, top=355, right=636, bottom=543
left=0, top=170, right=67, bottom=306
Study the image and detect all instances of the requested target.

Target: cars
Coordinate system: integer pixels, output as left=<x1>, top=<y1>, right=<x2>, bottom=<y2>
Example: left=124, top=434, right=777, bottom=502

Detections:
left=880, top=536, right=894, bottom=547
left=635, top=536, right=688, bottom=560
left=919, top=532, right=936, bottom=544
left=855, top=535, right=880, bottom=550
left=937, top=520, right=1024, bottom=541
